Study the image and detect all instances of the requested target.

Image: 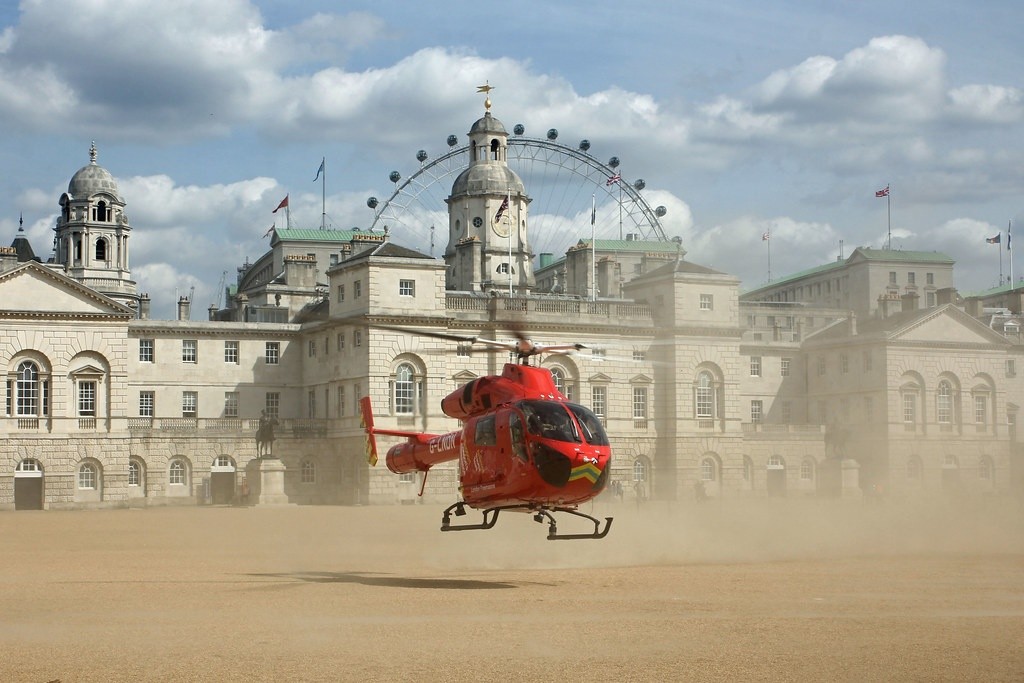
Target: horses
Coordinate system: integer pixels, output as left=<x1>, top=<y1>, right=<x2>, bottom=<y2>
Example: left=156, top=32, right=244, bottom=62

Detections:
left=255, top=415, right=280, bottom=461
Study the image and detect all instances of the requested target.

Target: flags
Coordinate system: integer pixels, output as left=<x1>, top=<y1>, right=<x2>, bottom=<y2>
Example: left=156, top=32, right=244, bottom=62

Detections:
left=985, top=234, right=1000, bottom=244
left=606, top=171, right=621, bottom=186
left=313, top=161, right=323, bottom=182
left=273, top=196, right=288, bottom=213
left=875, top=186, right=890, bottom=197
left=262, top=224, right=274, bottom=239
left=495, top=195, right=508, bottom=223
left=762, top=232, right=769, bottom=241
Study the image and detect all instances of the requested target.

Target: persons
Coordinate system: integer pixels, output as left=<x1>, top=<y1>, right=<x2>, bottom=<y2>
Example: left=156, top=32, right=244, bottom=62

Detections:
left=611, top=476, right=646, bottom=511
left=258, top=409, right=269, bottom=435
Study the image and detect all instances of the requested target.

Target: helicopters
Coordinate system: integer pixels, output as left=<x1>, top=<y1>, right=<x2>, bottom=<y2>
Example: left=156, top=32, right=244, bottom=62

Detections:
left=296, top=299, right=678, bottom=539
